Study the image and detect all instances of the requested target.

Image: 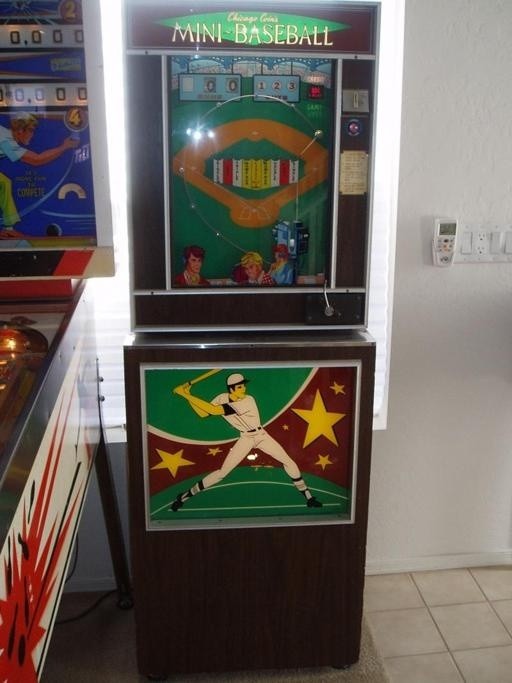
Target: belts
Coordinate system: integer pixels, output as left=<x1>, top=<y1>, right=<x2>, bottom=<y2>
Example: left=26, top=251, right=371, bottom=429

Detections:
left=240, top=426, right=261, bottom=433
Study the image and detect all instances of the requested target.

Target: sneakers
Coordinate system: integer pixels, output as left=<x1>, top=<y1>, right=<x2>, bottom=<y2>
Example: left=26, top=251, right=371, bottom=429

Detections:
left=307, top=497, right=322, bottom=508
left=0, top=229, right=25, bottom=237
left=171, top=494, right=183, bottom=512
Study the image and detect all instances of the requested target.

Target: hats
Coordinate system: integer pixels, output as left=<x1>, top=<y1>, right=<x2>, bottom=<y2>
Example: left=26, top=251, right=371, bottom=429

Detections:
left=227, top=374, right=249, bottom=385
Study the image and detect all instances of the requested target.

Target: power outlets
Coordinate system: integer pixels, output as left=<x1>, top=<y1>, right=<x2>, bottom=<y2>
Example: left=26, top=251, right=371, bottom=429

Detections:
left=472, top=226, right=491, bottom=264
